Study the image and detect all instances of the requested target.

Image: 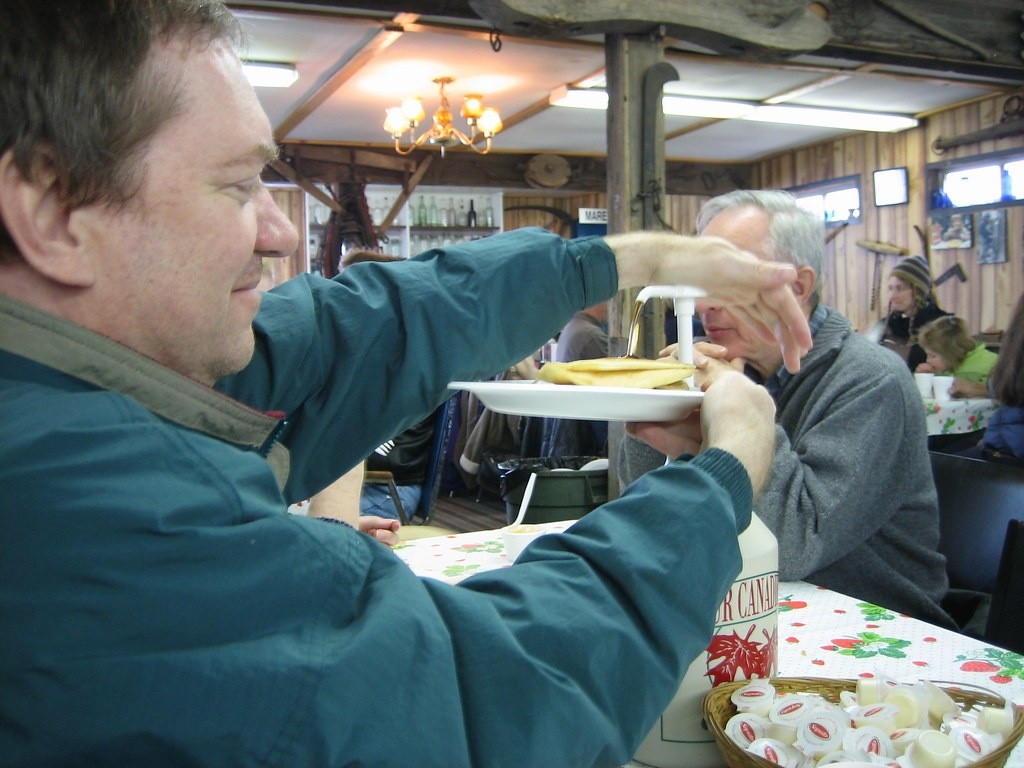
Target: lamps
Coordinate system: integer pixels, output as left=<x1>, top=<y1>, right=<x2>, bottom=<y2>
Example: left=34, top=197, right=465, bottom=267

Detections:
left=241, top=60, right=300, bottom=88
left=548, top=82, right=918, bottom=134
left=381, top=77, right=504, bottom=155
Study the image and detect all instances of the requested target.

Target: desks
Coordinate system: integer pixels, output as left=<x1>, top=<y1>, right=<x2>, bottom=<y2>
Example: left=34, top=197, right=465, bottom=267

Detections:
left=390, top=519, right=1024, bottom=768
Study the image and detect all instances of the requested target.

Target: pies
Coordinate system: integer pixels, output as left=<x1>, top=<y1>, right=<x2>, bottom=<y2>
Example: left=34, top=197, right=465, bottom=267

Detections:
left=537, top=356, right=696, bottom=389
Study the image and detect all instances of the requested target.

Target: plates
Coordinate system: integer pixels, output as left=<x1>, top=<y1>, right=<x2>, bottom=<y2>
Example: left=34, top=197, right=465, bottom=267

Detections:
left=446, top=380, right=707, bottom=422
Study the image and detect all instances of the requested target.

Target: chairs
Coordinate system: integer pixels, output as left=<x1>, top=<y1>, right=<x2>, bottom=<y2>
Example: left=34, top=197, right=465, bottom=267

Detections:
left=945, top=519, right=1024, bottom=655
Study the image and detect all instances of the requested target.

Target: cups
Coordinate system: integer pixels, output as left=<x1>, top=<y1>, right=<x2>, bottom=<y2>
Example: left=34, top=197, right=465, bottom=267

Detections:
left=933, top=377, right=954, bottom=402
left=914, top=373, right=934, bottom=398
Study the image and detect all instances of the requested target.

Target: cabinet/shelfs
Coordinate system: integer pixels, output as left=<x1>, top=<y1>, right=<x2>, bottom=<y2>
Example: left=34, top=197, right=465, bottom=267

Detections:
left=302, top=183, right=505, bottom=277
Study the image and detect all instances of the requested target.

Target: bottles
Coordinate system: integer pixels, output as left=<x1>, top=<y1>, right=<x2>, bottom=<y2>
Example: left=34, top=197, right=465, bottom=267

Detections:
left=478, top=196, right=486, bottom=226
left=448, top=198, right=456, bottom=227
left=469, top=199, right=476, bottom=227
left=308, top=239, right=317, bottom=258
left=310, top=257, right=321, bottom=277
left=411, top=232, right=490, bottom=257
left=1002, top=170, right=1011, bottom=200
left=418, top=195, right=427, bottom=227
left=439, top=197, right=448, bottom=227
left=932, top=188, right=950, bottom=208
left=367, top=195, right=399, bottom=224
left=429, top=196, right=438, bottom=226
left=407, top=198, right=415, bottom=225
left=831, top=210, right=835, bottom=221
left=392, top=239, right=401, bottom=255
left=308, top=196, right=330, bottom=223
left=457, top=198, right=467, bottom=226
left=486, top=197, right=494, bottom=227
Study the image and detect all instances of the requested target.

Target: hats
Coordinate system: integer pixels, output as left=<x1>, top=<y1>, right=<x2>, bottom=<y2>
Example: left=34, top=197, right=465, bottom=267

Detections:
left=890, top=255, right=931, bottom=295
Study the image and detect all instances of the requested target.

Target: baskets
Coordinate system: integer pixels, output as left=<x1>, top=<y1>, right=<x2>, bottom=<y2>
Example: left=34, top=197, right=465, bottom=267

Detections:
left=699, top=676, right=1024, bottom=768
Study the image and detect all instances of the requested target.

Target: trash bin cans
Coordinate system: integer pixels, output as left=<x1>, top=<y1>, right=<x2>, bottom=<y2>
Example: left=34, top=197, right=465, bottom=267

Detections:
left=497, top=455, right=608, bottom=526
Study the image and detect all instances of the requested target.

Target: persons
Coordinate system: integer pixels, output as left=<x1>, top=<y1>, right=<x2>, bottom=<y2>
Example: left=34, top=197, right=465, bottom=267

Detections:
left=615, top=189, right=961, bottom=634
left=852, top=256, right=1024, bottom=458
left=287, top=249, right=436, bottom=544
left=0, top=0, right=812, bottom=768
left=556, top=299, right=609, bottom=363
